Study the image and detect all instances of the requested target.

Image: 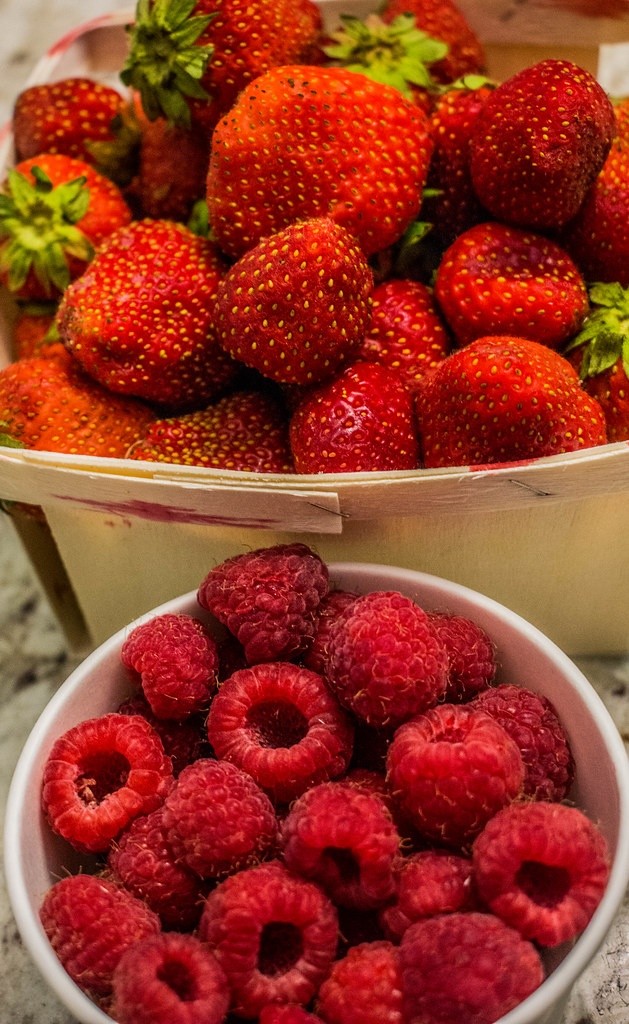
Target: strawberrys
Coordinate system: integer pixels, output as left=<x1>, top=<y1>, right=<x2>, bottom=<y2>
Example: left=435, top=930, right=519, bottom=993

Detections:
left=1, top=1, right=628, bottom=472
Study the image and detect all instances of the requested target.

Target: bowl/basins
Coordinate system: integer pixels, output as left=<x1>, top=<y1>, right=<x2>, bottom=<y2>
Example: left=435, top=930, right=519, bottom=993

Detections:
left=3, top=562, right=629, bottom=1024
